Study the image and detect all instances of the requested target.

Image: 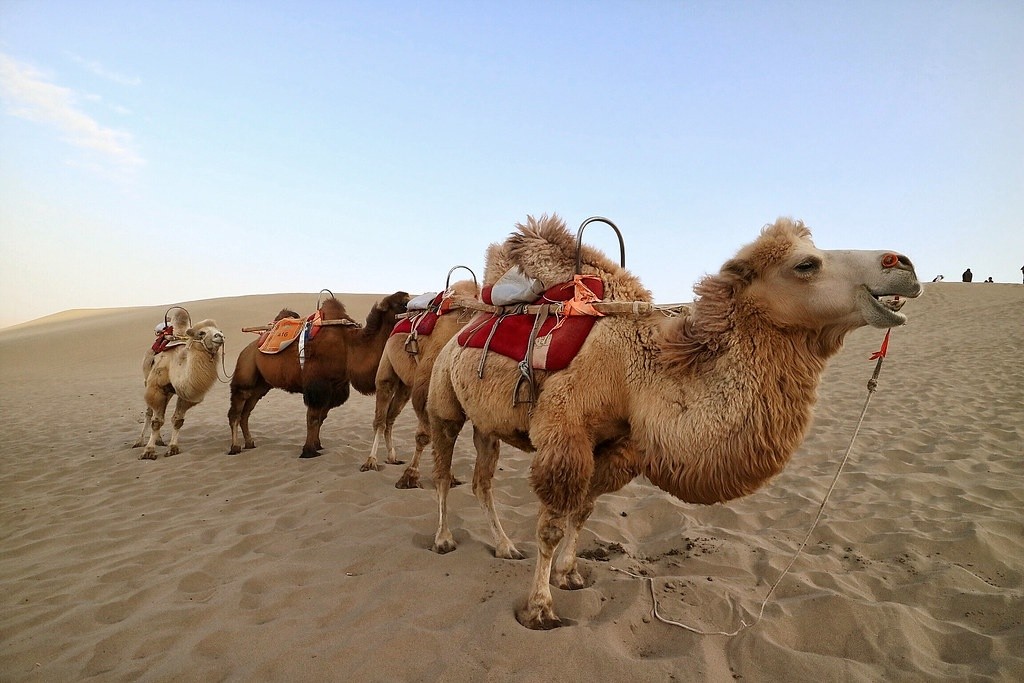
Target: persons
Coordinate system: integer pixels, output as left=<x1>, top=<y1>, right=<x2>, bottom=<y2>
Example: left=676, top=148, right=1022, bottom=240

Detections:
left=933, top=275, right=943, bottom=282
left=962, top=268, right=972, bottom=282
left=1020, top=266, right=1024, bottom=284
left=985, top=277, right=993, bottom=283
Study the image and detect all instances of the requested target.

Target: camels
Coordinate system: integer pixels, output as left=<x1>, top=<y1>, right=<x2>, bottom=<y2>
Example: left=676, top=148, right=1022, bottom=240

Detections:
left=227, top=290, right=413, bottom=459
left=129, top=305, right=227, bottom=462
left=358, top=213, right=925, bottom=632
